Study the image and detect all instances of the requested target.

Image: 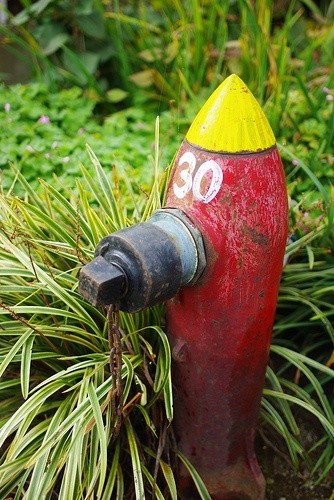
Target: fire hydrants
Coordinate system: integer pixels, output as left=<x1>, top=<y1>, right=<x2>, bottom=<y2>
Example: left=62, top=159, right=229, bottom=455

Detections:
left=75, top=74, right=292, bottom=496
